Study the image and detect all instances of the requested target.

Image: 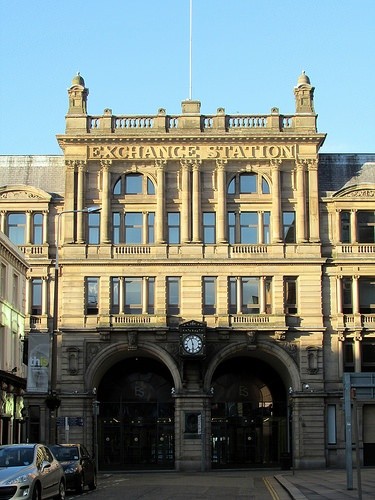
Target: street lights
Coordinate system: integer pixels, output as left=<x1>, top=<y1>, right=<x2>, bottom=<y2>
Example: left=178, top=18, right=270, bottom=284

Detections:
left=48, top=205, right=104, bottom=446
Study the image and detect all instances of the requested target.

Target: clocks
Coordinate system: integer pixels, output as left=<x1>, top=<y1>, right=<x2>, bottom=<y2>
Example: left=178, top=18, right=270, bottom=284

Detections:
left=179, top=319, right=207, bottom=360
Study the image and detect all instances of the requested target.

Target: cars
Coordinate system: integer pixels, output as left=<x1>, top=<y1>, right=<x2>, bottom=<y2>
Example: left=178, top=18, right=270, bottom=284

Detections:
left=49, top=442, right=97, bottom=495
left=0, top=443, right=68, bottom=500
